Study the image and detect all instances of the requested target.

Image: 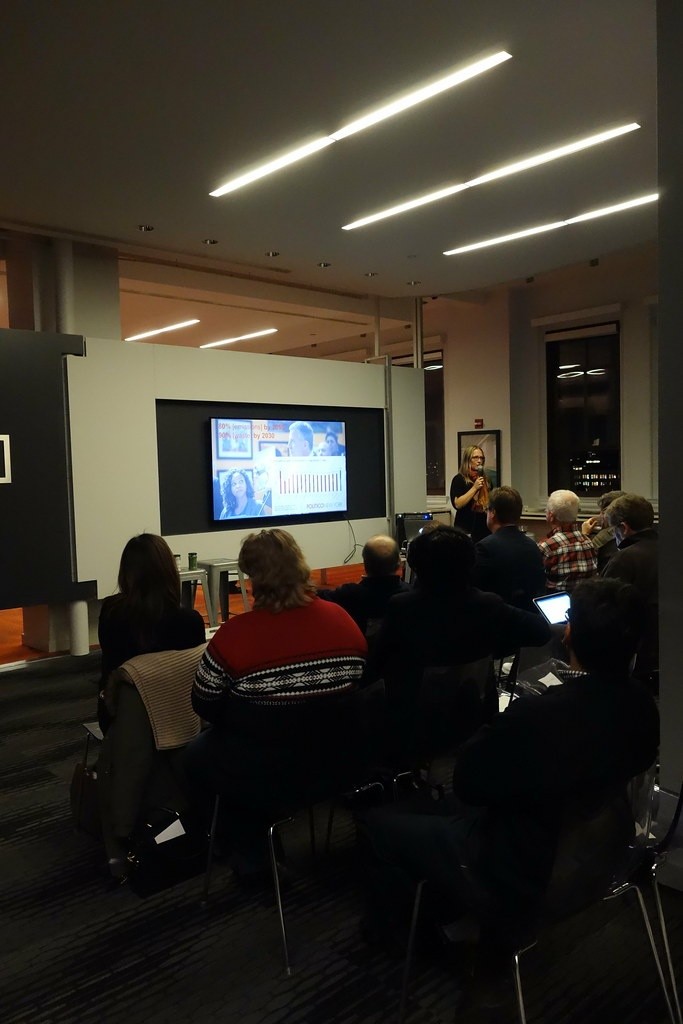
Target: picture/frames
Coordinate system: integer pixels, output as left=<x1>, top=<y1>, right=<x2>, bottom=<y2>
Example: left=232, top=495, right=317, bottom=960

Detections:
left=458, top=430, right=500, bottom=489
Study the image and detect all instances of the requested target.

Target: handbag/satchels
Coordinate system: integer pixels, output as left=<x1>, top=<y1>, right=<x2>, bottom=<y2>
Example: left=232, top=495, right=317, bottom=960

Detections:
left=70, top=732, right=107, bottom=825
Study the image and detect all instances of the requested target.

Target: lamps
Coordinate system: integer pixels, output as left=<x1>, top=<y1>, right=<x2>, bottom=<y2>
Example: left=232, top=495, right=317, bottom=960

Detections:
left=326, top=43, right=514, bottom=142
left=464, top=117, right=643, bottom=187
left=443, top=217, right=569, bottom=256
left=341, top=177, right=472, bottom=231
left=208, top=127, right=336, bottom=198
left=563, top=188, right=660, bottom=226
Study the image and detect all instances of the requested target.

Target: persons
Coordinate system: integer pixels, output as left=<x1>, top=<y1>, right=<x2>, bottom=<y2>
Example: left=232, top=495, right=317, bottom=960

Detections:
left=190, top=529, right=369, bottom=723
left=98, top=535, right=205, bottom=737
left=314, top=446, right=659, bottom=667
left=221, top=466, right=272, bottom=520
left=288, top=421, right=345, bottom=456
left=452, top=576, right=661, bottom=806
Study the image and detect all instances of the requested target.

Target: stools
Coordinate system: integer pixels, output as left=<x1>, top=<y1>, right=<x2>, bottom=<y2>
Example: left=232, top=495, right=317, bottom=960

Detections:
left=193, top=559, right=250, bottom=627
left=176, top=565, right=213, bottom=628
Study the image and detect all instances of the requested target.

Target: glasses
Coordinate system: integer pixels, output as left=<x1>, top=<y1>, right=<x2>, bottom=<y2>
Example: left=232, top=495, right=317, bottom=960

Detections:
left=471, top=456, right=485, bottom=461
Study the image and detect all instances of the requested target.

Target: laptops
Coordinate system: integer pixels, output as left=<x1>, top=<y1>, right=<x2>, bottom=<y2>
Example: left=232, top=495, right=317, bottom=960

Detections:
left=532, top=591, right=570, bottom=625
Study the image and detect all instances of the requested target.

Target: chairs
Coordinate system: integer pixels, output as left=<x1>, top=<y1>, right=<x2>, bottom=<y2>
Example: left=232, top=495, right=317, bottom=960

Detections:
left=491, top=563, right=547, bottom=708
left=320, top=643, right=525, bottom=856
left=628, top=602, right=658, bottom=697
left=634, top=783, right=683, bottom=1024
left=397, top=748, right=677, bottom=1024
left=76, top=641, right=213, bottom=905
left=365, top=589, right=408, bottom=643
left=202, top=676, right=395, bottom=978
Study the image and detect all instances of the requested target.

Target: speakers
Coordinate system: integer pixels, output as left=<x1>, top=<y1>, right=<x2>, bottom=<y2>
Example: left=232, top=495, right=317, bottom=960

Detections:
left=396, top=513, right=433, bottom=551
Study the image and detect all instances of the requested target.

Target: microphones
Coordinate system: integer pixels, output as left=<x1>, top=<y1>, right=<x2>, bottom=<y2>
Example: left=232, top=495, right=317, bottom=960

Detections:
left=478, top=465, right=483, bottom=477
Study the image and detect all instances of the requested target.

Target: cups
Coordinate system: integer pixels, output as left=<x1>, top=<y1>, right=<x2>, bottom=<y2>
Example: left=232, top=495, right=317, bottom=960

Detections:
left=519, top=526, right=527, bottom=533
left=188, top=553, right=197, bottom=570
left=174, top=555, right=181, bottom=571
